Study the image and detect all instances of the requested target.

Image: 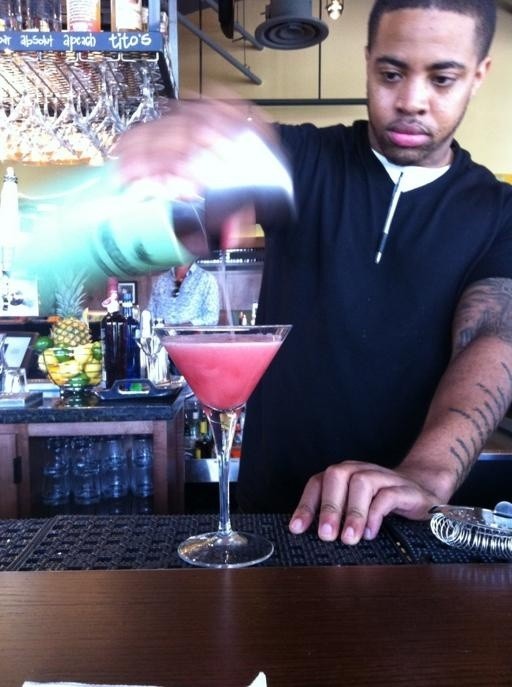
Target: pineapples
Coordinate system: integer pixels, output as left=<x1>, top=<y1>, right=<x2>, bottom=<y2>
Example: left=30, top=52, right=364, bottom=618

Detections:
left=50, top=268, right=93, bottom=348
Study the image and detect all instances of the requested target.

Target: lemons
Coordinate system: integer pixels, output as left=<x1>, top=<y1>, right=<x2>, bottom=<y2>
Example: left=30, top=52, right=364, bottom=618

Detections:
left=44, top=342, right=102, bottom=388
left=34, top=336, right=52, bottom=353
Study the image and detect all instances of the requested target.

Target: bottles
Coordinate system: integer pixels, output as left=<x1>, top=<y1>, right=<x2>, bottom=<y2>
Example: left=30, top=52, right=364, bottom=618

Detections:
left=102, top=277, right=169, bottom=387
left=182, top=403, right=243, bottom=459
left=0, top=0, right=146, bottom=32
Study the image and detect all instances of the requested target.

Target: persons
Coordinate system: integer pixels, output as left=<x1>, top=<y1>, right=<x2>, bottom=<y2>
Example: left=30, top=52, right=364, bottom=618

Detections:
left=112, top=0, right=511, bottom=548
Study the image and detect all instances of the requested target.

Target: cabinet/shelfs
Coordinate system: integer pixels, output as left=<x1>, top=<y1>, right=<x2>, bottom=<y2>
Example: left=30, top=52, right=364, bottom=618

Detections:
left=0, top=386, right=184, bottom=519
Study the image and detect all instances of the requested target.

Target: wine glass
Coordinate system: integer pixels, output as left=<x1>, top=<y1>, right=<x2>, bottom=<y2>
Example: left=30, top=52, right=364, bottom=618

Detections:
left=0, top=52, right=164, bottom=159
left=155, top=324, right=294, bottom=569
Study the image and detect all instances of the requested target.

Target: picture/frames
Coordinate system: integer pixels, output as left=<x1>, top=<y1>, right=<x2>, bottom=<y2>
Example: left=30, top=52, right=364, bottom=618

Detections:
left=117, top=280, right=138, bottom=306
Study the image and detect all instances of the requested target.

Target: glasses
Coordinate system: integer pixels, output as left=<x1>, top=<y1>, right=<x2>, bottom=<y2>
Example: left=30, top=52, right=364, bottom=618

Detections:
left=172, top=280, right=182, bottom=297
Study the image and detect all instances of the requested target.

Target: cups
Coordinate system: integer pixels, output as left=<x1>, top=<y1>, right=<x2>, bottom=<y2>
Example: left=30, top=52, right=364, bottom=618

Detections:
left=42, top=437, right=154, bottom=504
left=3, top=367, right=29, bottom=398
